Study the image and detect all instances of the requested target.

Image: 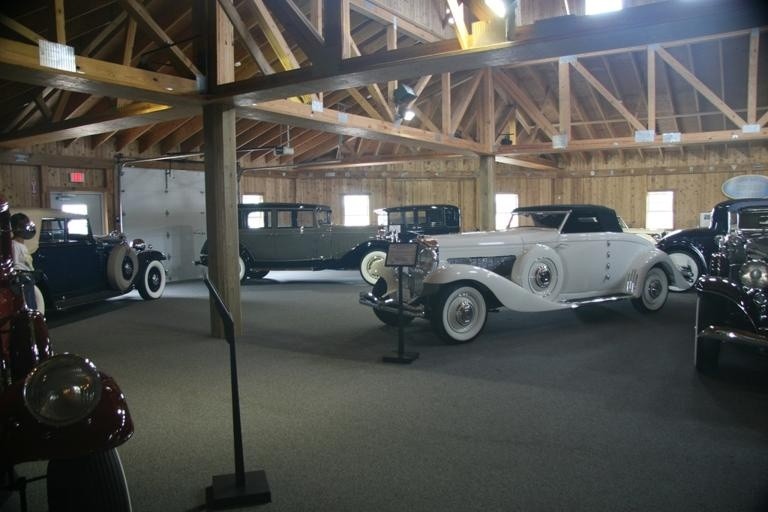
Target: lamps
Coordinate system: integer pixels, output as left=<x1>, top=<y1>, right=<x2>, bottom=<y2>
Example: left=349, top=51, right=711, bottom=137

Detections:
left=391, top=85, right=418, bottom=127
left=484, top=0, right=519, bottom=41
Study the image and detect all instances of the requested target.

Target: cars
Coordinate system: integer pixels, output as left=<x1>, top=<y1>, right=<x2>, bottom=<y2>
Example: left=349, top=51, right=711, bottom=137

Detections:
left=1, top=203, right=167, bottom=512
